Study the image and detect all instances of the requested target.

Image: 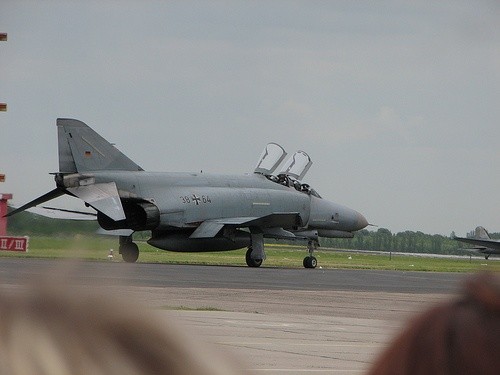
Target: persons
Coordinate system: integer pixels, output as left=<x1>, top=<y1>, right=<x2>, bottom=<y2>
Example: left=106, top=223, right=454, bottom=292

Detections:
left=364, top=271, right=500, bottom=375
left=0, top=282, right=245, bottom=375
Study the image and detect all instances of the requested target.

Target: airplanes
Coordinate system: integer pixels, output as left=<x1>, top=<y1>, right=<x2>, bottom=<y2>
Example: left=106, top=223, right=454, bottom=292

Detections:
left=453, top=227, right=500, bottom=260
left=2, top=118, right=379, bottom=268
